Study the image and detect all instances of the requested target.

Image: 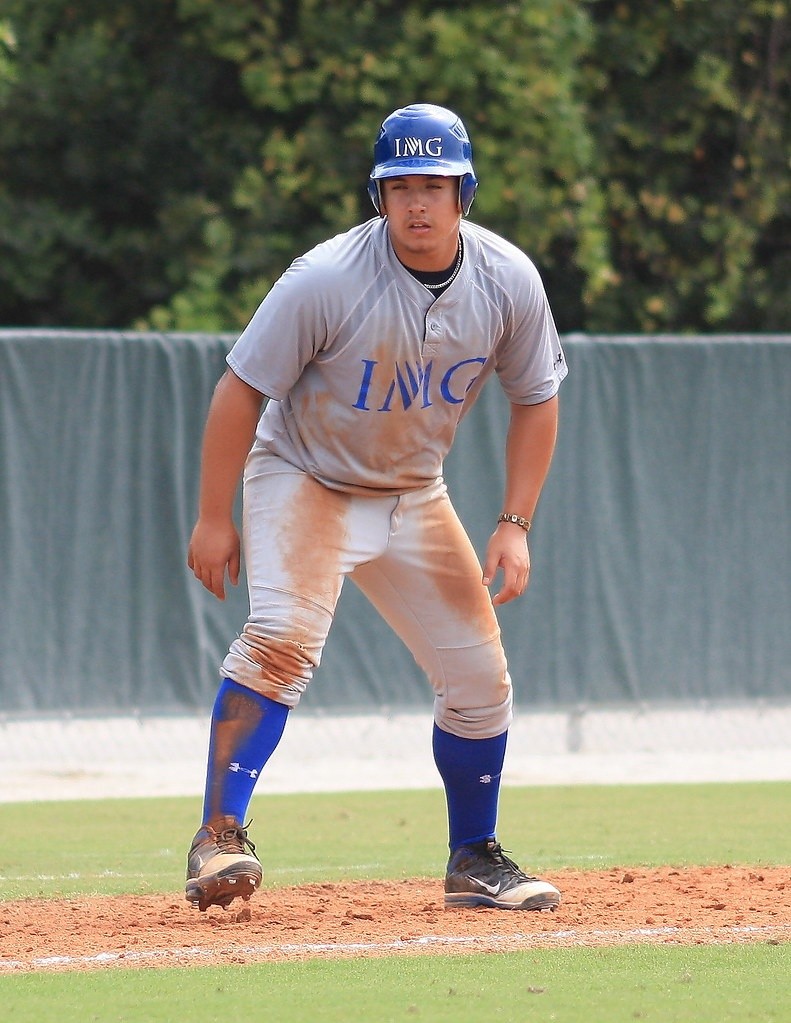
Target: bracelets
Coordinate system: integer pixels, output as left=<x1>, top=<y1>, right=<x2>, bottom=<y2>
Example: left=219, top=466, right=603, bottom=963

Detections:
left=497, top=513, right=531, bottom=531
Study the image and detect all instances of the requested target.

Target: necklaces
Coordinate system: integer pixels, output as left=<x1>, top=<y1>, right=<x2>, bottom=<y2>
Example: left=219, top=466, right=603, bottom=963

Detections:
left=425, top=236, right=462, bottom=289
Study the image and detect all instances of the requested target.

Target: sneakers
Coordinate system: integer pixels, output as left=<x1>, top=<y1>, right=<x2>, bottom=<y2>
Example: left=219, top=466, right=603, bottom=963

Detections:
left=186, top=815, right=263, bottom=912
left=444, top=837, right=561, bottom=912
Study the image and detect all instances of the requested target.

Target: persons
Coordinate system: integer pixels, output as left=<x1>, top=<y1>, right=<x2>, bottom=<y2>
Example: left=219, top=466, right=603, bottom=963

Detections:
left=186, top=102, right=570, bottom=912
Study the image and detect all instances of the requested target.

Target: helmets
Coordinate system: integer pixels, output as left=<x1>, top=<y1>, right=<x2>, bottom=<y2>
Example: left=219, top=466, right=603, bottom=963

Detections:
left=367, top=104, right=479, bottom=219
